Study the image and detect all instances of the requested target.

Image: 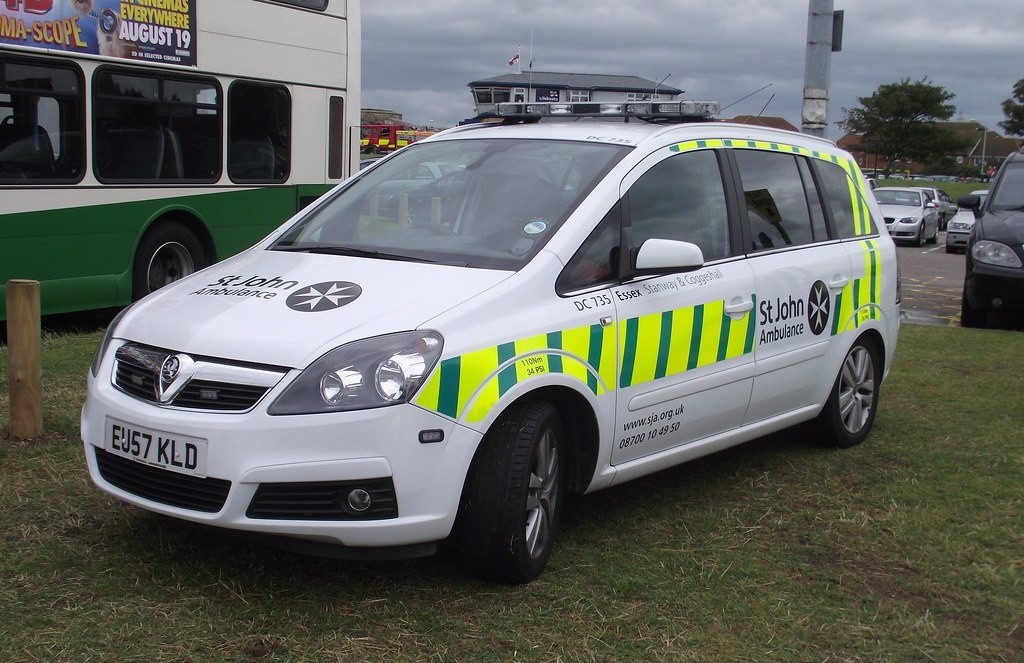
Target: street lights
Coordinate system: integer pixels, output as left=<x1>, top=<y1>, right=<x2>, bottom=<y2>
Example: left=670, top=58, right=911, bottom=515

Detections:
left=976, top=127, right=988, bottom=175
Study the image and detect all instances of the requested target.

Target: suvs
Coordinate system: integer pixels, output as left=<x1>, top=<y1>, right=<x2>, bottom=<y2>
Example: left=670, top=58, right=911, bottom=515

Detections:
left=959, top=145, right=1024, bottom=329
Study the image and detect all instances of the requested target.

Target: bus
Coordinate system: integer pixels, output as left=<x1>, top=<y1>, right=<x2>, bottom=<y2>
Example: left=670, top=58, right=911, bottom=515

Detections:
left=0, top=1, right=360, bottom=322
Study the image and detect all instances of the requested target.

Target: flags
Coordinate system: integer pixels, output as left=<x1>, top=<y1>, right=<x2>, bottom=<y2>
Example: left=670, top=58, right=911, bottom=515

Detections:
left=508, top=53, right=519, bottom=66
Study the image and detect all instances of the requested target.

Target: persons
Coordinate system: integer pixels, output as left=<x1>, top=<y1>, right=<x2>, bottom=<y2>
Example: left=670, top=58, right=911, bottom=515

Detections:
left=986, top=165, right=997, bottom=177
left=882, top=167, right=886, bottom=175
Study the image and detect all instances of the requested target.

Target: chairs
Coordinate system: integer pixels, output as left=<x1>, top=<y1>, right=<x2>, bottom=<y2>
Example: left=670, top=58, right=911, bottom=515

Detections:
left=0, top=116, right=277, bottom=179
left=472, top=174, right=553, bottom=255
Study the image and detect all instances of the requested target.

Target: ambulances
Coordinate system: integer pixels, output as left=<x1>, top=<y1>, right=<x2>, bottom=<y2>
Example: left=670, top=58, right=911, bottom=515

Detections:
left=80, top=99, right=903, bottom=587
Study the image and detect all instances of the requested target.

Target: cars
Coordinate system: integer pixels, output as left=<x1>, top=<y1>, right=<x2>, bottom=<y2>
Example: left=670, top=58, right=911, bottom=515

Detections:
left=945, top=189, right=989, bottom=253
left=863, top=174, right=877, bottom=191
left=871, top=187, right=940, bottom=249
left=895, top=187, right=959, bottom=232
